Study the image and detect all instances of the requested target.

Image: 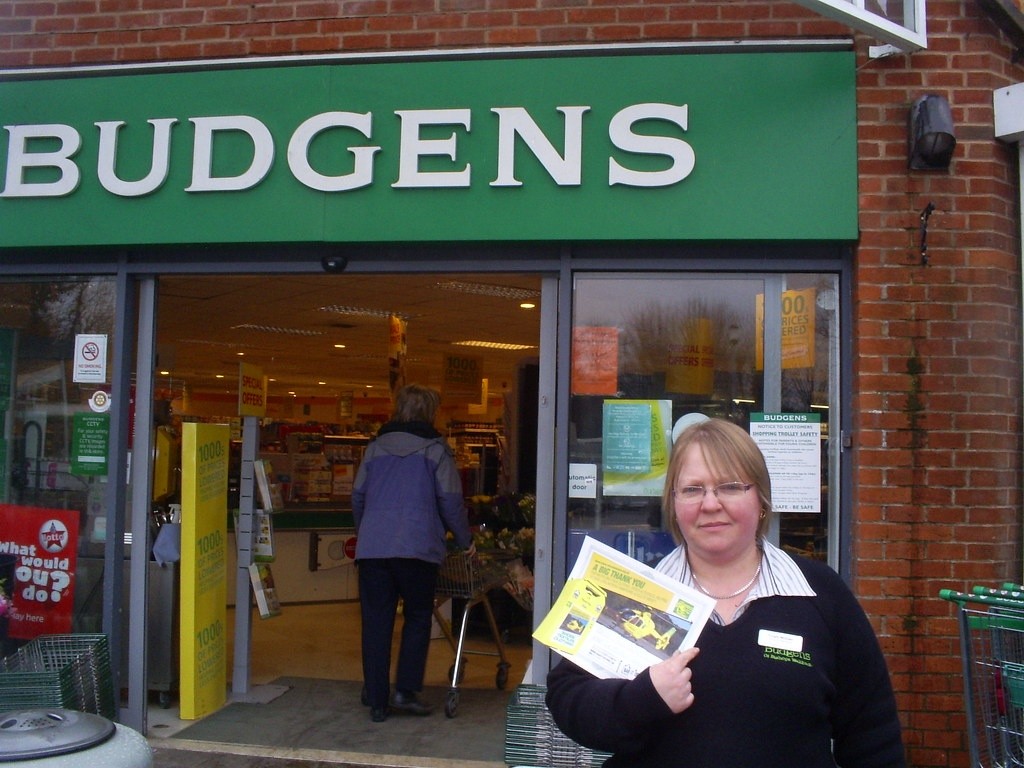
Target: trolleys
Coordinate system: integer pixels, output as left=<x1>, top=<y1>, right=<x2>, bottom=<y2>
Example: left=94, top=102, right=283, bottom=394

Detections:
left=938, top=582, right=1024, bottom=768
left=432, top=544, right=512, bottom=718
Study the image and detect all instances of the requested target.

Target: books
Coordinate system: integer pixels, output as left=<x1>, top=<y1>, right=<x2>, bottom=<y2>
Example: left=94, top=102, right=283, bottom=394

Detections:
left=231, top=455, right=290, bottom=621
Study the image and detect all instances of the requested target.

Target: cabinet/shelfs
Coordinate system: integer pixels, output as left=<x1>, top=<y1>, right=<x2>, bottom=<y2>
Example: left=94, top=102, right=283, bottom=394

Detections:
left=226, top=415, right=293, bottom=706
left=169, top=355, right=541, bottom=509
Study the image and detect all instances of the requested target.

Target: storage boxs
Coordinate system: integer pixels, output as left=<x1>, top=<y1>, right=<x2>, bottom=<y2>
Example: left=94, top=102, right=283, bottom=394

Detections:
left=430, top=596, right=453, bottom=640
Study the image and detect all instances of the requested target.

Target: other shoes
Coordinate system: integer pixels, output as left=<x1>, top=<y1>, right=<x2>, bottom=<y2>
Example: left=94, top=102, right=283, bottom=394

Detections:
left=390, top=691, right=434, bottom=716
left=372, top=704, right=392, bottom=722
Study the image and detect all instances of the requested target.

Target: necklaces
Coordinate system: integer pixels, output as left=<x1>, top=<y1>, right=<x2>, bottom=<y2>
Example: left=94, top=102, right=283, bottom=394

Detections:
left=690, top=553, right=763, bottom=602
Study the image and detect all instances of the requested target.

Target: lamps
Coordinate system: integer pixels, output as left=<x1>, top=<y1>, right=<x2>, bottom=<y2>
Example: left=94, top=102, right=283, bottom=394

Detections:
left=906, top=90, right=957, bottom=175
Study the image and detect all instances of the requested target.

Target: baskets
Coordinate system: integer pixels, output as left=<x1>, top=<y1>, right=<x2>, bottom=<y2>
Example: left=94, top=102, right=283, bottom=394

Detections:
left=0, top=633, right=121, bottom=724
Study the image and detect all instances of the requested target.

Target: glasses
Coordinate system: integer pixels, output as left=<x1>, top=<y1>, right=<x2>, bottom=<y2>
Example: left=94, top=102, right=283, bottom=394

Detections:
left=672, top=482, right=757, bottom=504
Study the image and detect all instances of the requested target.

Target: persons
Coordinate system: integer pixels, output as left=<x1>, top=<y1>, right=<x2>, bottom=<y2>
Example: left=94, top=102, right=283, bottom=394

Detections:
left=348, top=384, right=477, bottom=723
left=538, top=413, right=911, bottom=768
